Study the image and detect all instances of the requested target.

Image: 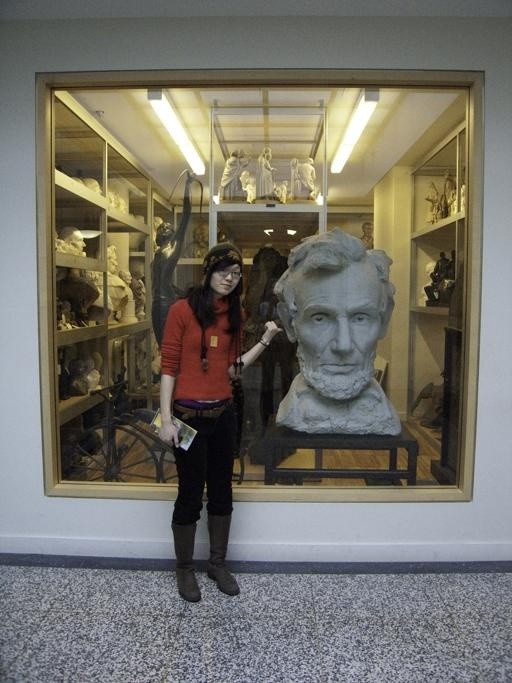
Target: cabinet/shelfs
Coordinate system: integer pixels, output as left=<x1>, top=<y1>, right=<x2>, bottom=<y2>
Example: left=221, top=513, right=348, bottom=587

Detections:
left=51, top=88, right=210, bottom=427
left=205, top=96, right=330, bottom=259
left=402, top=119, right=468, bottom=474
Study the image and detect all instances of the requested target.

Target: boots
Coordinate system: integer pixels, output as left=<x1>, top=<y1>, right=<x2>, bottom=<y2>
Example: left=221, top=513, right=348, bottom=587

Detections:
left=171, top=523, right=200, bottom=602
left=206, top=513, right=240, bottom=594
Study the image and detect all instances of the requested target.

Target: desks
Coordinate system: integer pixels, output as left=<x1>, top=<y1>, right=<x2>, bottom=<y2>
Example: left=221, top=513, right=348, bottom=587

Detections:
left=260, top=410, right=421, bottom=488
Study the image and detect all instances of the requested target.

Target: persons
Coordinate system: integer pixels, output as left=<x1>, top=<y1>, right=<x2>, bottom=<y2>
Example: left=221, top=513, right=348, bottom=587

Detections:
left=274, top=226, right=402, bottom=435
left=421, top=168, right=467, bottom=307
left=260, top=331, right=293, bottom=428
left=216, top=146, right=320, bottom=206
left=151, top=171, right=196, bottom=347
left=55, top=162, right=163, bottom=482
left=161, top=242, right=283, bottom=603
left=360, top=222, right=375, bottom=250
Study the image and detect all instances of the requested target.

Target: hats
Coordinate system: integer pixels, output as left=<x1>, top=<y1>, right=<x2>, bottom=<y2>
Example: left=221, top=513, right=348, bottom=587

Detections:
left=202, top=242, right=243, bottom=273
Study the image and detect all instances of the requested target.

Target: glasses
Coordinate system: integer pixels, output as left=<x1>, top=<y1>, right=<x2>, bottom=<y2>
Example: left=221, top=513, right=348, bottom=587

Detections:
left=218, top=267, right=242, bottom=278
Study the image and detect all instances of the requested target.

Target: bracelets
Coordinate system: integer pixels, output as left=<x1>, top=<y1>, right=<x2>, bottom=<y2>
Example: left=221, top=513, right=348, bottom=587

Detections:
left=260, top=336, right=270, bottom=351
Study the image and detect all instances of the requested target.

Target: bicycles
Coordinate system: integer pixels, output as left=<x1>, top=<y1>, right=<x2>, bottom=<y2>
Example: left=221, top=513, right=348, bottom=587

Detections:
left=63, top=376, right=245, bottom=486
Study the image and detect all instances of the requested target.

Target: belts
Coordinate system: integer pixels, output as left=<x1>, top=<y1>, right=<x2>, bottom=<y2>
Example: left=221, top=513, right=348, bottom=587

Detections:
left=174, top=404, right=230, bottom=419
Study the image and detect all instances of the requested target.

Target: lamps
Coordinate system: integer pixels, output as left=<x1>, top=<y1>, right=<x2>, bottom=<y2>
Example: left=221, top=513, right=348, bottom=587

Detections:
left=329, top=89, right=383, bottom=174
